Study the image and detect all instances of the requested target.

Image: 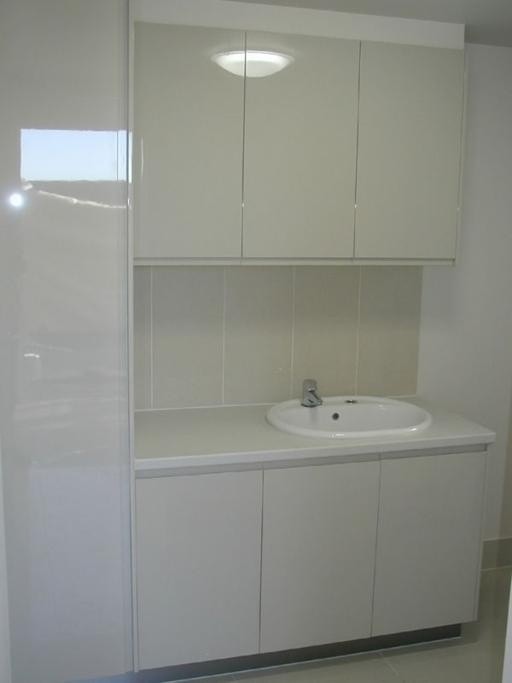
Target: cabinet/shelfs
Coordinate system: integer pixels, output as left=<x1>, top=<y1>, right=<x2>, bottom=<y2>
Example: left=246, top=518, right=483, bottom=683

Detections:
left=131, top=19, right=468, bottom=266
left=134, top=443, right=488, bottom=682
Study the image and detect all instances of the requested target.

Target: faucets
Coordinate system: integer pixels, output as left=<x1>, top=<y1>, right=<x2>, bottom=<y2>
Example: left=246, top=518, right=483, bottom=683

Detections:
left=301, top=378, right=323, bottom=408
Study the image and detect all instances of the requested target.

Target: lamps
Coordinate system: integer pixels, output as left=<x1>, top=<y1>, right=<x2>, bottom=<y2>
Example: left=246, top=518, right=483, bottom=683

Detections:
left=209, top=51, right=294, bottom=79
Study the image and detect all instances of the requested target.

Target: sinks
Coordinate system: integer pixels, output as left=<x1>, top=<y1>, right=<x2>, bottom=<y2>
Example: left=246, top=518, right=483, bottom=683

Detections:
left=265, top=395, right=435, bottom=439
